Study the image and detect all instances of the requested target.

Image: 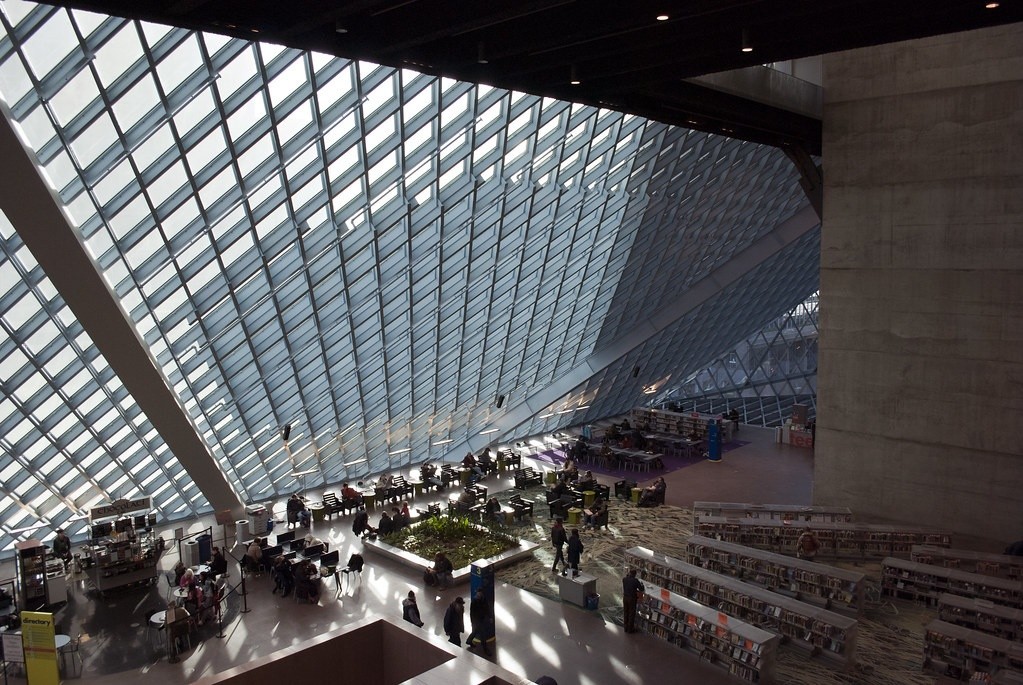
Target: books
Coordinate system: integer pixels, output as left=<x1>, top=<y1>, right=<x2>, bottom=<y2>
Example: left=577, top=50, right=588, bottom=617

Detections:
left=883, top=554, right=1023, bottom=670
left=625, top=544, right=856, bottom=652
left=633, top=590, right=762, bottom=682
left=637, top=410, right=707, bottom=437
left=694, top=508, right=949, bottom=552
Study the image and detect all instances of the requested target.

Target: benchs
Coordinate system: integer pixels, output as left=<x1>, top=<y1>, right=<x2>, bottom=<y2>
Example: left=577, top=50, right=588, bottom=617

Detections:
left=514, top=466, right=543, bottom=490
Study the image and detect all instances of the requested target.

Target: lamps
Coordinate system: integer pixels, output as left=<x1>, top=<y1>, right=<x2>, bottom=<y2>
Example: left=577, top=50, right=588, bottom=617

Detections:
left=497, top=394, right=505, bottom=409
left=282, top=424, right=291, bottom=441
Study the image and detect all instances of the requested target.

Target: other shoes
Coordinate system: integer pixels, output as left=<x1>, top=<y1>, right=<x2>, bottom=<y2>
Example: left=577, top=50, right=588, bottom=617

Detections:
left=562, top=570, right=567, bottom=576
left=483, top=645, right=492, bottom=656
left=466, top=641, right=476, bottom=647
left=624, top=629, right=628, bottom=632
left=628, top=627, right=639, bottom=634
left=552, top=568, right=559, bottom=572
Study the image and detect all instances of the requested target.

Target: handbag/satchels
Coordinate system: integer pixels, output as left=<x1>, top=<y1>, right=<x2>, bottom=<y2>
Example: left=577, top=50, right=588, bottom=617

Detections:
left=424, top=571, right=433, bottom=585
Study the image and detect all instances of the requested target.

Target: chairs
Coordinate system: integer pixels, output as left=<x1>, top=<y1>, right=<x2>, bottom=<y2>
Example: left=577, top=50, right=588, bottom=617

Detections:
left=62, top=632, right=85, bottom=679
left=546, top=468, right=666, bottom=531
left=508, top=493, right=535, bottom=521
left=144, top=555, right=228, bottom=657
left=286, top=506, right=311, bottom=528
left=322, top=449, right=522, bottom=521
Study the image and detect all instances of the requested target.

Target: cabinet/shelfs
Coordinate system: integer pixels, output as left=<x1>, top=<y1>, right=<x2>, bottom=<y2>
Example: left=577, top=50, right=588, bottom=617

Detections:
left=623, top=500, right=1023, bottom=685
left=14, top=539, right=50, bottom=616
left=246, top=505, right=269, bottom=536
left=45, top=558, right=68, bottom=607
left=630, top=406, right=734, bottom=445
left=556, top=568, right=599, bottom=608
left=83, top=528, right=167, bottom=595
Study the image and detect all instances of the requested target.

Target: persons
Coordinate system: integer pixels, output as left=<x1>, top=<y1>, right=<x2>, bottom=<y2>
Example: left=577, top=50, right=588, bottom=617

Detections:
left=248, top=537, right=262, bottom=561
left=304, top=533, right=327, bottom=552
left=402, top=591, right=424, bottom=628
left=575, top=418, right=650, bottom=455
left=552, top=518, right=583, bottom=573
left=287, top=493, right=311, bottom=526
left=273, top=555, right=309, bottom=598
left=444, top=596, right=465, bottom=647
left=429, top=551, right=452, bottom=591
left=796, top=526, right=821, bottom=562
left=552, top=457, right=606, bottom=532
left=342, top=447, right=508, bottom=530
left=730, top=408, right=739, bottom=431
left=54, top=530, right=74, bottom=565
left=181, top=546, right=225, bottom=625
left=621, top=570, right=644, bottom=634
left=637, top=477, right=666, bottom=507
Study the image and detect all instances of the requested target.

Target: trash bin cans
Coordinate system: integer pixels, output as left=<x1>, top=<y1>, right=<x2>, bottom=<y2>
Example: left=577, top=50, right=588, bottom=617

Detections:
left=587, top=593, right=600, bottom=610
left=195, top=534, right=211, bottom=565
left=775, top=426, right=782, bottom=443
left=185, top=540, right=199, bottom=567
left=235, top=520, right=249, bottom=545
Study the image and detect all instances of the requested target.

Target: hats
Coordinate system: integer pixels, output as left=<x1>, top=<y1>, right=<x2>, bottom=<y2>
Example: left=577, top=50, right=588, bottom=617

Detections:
left=455, top=597, right=465, bottom=604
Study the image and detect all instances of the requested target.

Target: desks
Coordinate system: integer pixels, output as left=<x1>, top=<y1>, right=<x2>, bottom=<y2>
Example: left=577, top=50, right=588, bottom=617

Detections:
left=618, top=429, right=703, bottom=459
left=148, top=610, right=166, bottom=652
left=172, top=585, right=204, bottom=608
left=496, top=460, right=506, bottom=471
left=581, top=491, right=596, bottom=506
left=563, top=437, right=663, bottom=473
left=55, top=634, right=71, bottom=681
left=567, top=508, right=581, bottom=526
left=186, top=565, right=211, bottom=583
left=241, top=533, right=355, bottom=597
left=547, top=470, right=557, bottom=484
left=630, top=487, right=643, bottom=502
left=411, top=481, right=423, bottom=497
left=312, top=505, right=325, bottom=523
left=459, top=468, right=472, bottom=483
left=362, top=492, right=376, bottom=509
